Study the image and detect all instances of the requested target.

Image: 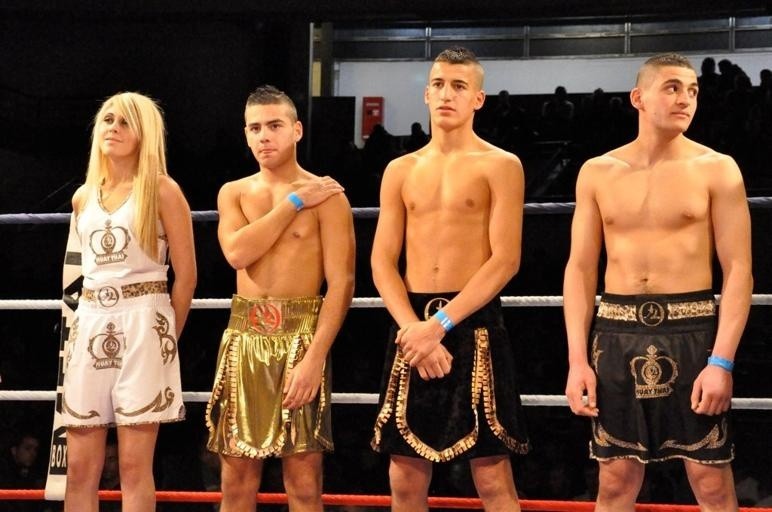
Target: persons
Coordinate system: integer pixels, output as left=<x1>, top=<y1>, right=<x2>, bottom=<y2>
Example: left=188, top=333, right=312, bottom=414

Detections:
left=0, top=54, right=772, bottom=487
left=369, top=46, right=531, bottom=512
left=204, top=82, right=359, bottom=512
left=58, top=90, right=199, bottom=511
left=561, top=50, right=755, bottom=512
